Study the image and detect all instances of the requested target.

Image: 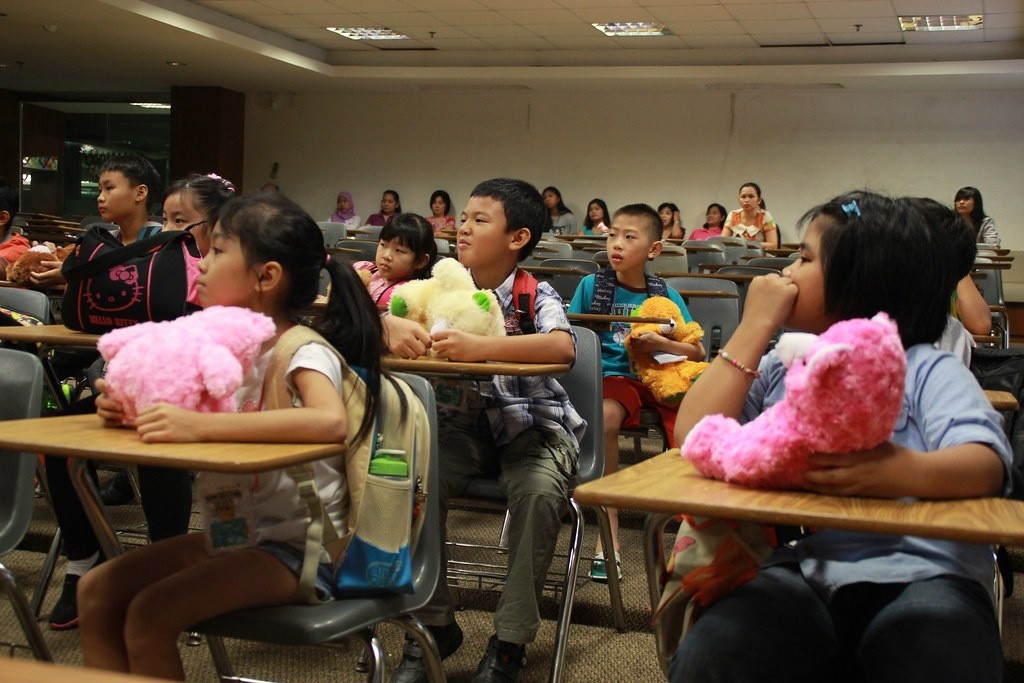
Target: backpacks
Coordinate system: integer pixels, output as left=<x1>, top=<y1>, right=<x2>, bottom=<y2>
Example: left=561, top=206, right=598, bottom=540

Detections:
left=0, top=305, right=87, bottom=412
left=262, top=321, right=431, bottom=605
left=967, top=346, right=1024, bottom=503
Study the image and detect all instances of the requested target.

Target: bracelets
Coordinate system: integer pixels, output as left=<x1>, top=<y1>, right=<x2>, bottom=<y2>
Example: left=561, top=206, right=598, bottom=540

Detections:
left=673, top=220, right=681, bottom=224
left=717, top=347, right=764, bottom=379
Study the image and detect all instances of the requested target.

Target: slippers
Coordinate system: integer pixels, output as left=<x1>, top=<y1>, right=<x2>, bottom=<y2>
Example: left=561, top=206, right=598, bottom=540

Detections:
left=587, top=550, right=622, bottom=583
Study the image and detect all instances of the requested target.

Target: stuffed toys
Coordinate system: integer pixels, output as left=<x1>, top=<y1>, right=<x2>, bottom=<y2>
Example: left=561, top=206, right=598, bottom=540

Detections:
left=386, top=257, right=506, bottom=358
left=95, top=304, right=278, bottom=426
left=624, top=295, right=711, bottom=409
left=6, top=240, right=81, bottom=292
left=679, top=312, right=908, bottom=490
left=314, top=269, right=372, bottom=304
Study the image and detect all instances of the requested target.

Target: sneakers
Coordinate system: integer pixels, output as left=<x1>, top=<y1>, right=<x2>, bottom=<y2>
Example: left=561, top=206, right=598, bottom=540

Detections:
left=472, top=634, right=528, bottom=683
left=391, top=621, right=463, bottom=683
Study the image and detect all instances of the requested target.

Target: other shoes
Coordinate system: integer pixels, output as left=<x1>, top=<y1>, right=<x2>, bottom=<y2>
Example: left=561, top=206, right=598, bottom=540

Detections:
left=100, top=470, right=137, bottom=505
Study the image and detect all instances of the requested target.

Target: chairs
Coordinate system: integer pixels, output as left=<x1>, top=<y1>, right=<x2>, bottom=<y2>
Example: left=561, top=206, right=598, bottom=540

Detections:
left=0, top=218, right=1024, bottom=683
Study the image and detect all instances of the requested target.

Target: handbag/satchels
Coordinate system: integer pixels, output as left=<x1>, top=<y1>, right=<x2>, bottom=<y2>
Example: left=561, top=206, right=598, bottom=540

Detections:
left=651, top=512, right=775, bottom=680
left=48, top=551, right=108, bottom=631
left=60, top=218, right=209, bottom=339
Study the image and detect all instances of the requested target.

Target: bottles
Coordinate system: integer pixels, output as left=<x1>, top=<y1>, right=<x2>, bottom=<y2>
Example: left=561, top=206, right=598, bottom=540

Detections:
left=368, top=449, right=407, bottom=482
left=549, top=229, right=560, bottom=235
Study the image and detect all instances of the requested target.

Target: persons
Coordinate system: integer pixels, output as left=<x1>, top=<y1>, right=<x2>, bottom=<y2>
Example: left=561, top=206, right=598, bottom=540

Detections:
left=78, top=192, right=410, bottom=682
left=314, top=213, right=438, bottom=319
left=377, top=178, right=587, bottom=683
left=950, top=274, right=993, bottom=337
left=953, top=186, right=999, bottom=250
left=161, top=171, right=237, bottom=258
left=542, top=182, right=779, bottom=251
left=563, top=203, right=707, bottom=585
left=898, top=195, right=971, bottom=370
left=667, top=190, right=1014, bottom=682
left=329, top=190, right=456, bottom=232
left=0, top=155, right=163, bottom=286
